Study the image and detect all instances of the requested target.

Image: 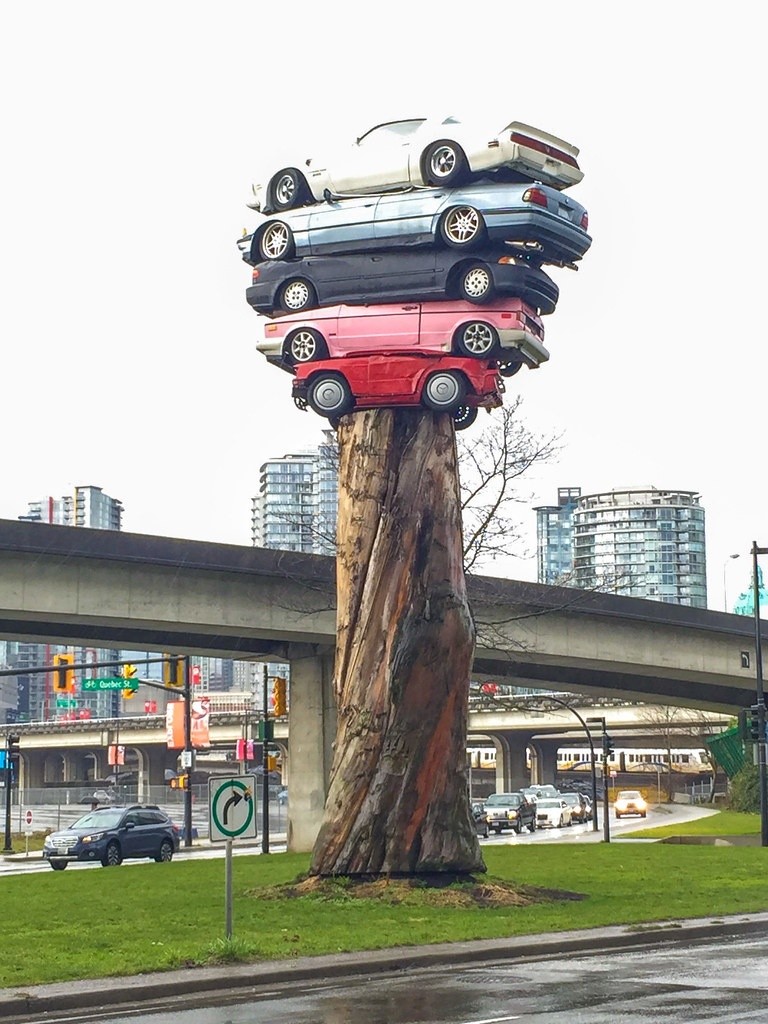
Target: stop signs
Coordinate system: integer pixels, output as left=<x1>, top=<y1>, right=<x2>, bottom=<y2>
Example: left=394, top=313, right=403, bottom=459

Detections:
left=25, top=810, right=33, bottom=824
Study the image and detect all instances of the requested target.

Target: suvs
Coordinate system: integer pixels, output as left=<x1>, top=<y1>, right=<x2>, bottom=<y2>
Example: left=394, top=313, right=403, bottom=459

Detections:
left=41, top=804, right=181, bottom=872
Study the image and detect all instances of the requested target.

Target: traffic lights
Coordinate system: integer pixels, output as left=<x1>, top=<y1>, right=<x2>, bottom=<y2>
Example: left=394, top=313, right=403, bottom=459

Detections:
left=750, top=703, right=768, bottom=739
left=179, top=776, right=189, bottom=789
left=191, top=665, right=202, bottom=684
left=7, top=733, right=20, bottom=763
left=143, top=700, right=150, bottom=714
left=152, top=701, right=157, bottom=713
left=78, top=709, right=86, bottom=719
left=170, top=777, right=180, bottom=789
left=602, top=733, right=615, bottom=755
left=270, top=675, right=285, bottom=715
left=121, top=664, right=139, bottom=700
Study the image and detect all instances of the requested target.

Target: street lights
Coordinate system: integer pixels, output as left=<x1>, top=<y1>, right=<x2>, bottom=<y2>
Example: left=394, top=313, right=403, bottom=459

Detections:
left=584, top=716, right=611, bottom=842
left=722, top=552, right=741, bottom=613
left=750, top=540, right=768, bottom=847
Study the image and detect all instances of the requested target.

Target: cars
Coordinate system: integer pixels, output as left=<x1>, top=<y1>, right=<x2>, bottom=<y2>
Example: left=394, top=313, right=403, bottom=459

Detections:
left=472, top=785, right=593, bottom=839
left=288, top=355, right=507, bottom=429
left=75, top=743, right=287, bottom=807
left=242, top=114, right=585, bottom=216
left=236, top=177, right=593, bottom=272
left=252, top=294, right=551, bottom=378
left=612, top=790, right=648, bottom=818
left=243, top=243, right=560, bottom=316
